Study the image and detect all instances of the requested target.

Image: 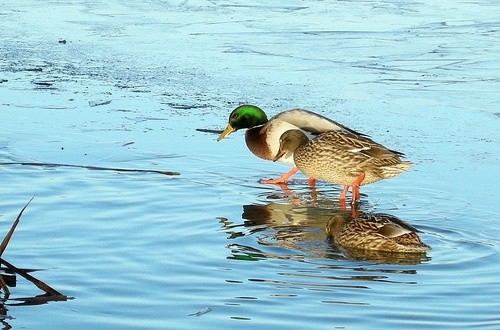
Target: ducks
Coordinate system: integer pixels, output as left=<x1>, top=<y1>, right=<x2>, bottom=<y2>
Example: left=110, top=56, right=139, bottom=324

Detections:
left=217, top=105, right=372, bottom=184
left=272, top=129, right=415, bottom=204
left=325, top=212, right=433, bottom=253
